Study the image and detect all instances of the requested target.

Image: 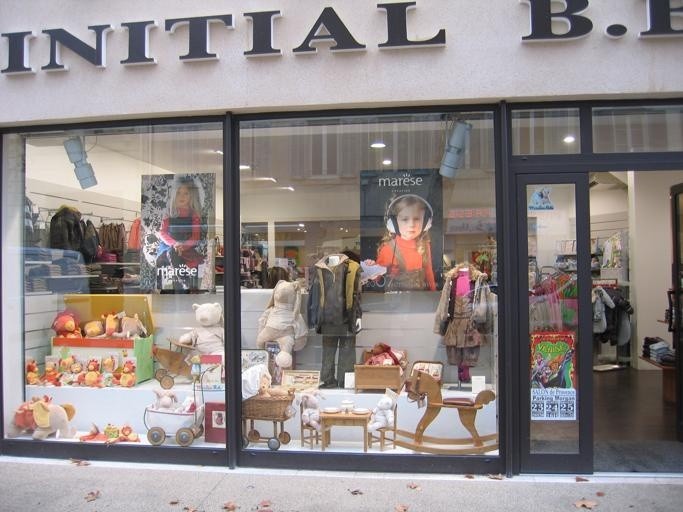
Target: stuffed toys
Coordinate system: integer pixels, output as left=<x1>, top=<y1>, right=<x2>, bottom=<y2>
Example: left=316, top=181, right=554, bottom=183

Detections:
left=259, top=373, right=296, bottom=398
left=3, top=305, right=149, bottom=447
left=180, top=299, right=226, bottom=384
left=364, top=394, right=395, bottom=433
left=299, top=391, right=330, bottom=434
left=254, top=279, right=309, bottom=371
left=151, top=389, right=198, bottom=414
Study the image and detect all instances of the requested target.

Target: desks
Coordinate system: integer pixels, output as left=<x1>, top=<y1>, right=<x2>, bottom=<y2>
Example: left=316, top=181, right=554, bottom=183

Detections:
left=320, top=411, right=372, bottom=452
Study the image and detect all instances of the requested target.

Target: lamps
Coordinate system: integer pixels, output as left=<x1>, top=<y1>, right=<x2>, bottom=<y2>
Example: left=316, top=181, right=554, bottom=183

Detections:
left=63, top=136, right=97, bottom=190
left=439, top=117, right=472, bottom=179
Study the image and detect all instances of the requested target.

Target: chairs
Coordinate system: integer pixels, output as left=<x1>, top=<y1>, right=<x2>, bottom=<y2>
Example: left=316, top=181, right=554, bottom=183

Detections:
left=300, top=402, right=322, bottom=449
left=368, top=404, right=397, bottom=451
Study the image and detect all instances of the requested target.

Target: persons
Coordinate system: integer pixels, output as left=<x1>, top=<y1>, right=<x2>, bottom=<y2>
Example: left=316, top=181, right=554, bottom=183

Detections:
left=532, top=351, right=545, bottom=382
left=151, top=175, right=209, bottom=293
left=433, top=262, right=492, bottom=385
left=541, top=359, right=563, bottom=388
left=306, top=252, right=363, bottom=390
left=363, top=190, right=438, bottom=293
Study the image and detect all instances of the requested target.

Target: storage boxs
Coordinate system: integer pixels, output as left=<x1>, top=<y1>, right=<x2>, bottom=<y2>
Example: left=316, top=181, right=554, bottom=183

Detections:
left=51, top=294, right=154, bottom=386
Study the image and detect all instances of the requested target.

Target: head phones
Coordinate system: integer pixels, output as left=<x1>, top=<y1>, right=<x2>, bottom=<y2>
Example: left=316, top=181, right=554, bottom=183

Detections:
left=384, top=193, right=434, bottom=234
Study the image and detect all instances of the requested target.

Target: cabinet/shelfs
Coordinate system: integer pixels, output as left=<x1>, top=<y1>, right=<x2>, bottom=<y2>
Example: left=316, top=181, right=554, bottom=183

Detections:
left=25, top=260, right=141, bottom=374
left=554, top=236, right=605, bottom=273
left=216, top=256, right=226, bottom=286
